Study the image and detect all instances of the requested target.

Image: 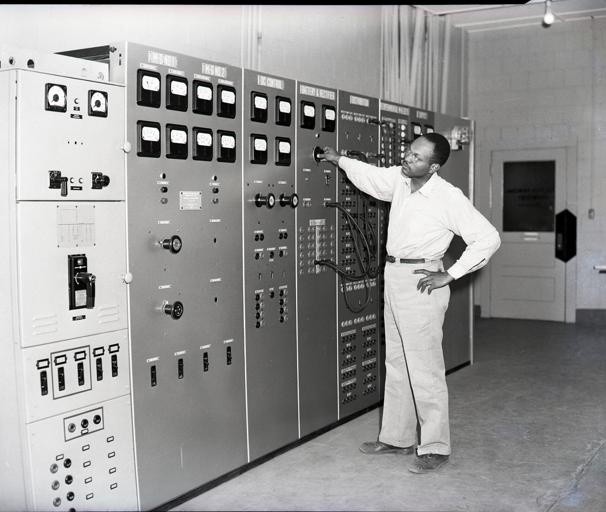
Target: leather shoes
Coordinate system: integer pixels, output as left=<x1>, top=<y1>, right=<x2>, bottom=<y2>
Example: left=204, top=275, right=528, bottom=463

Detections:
left=409, top=453, right=450, bottom=473
left=359, top=441, right=414, bottom=455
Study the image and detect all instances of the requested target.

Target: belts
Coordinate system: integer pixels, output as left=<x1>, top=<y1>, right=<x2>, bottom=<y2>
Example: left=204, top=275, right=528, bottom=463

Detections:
left=385, top=255, right=426, bottom=264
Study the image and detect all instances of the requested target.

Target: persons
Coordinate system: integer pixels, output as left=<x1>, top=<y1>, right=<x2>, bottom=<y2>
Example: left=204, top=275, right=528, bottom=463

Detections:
left=317, top=134, right=501, bottom=474
